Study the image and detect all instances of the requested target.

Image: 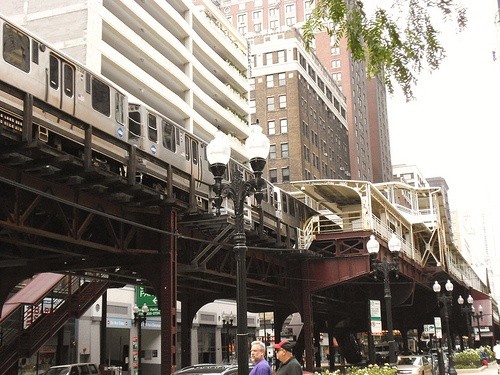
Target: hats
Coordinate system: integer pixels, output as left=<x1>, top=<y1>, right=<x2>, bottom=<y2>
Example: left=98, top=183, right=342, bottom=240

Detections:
left=272, top=339, right=293, bottom=352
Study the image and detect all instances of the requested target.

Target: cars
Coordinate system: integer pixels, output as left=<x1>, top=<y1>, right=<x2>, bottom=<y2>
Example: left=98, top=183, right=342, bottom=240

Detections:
left=394, top=355, right=434, bottom=375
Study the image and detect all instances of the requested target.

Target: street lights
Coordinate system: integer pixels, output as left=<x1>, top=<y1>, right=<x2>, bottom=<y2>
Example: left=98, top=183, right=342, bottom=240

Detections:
left=221, top=310, right=234, bottom=364
left=203, top=127, right=273, bottom=371
left=470, top=303, right=484, bottom=343
left=366, top=231, right=403, bottom=366
left=433, top=277, right=456, bottom=371
left=132, top=303, right=149, bottom=375
left=457, top=288, right=474, bottom=347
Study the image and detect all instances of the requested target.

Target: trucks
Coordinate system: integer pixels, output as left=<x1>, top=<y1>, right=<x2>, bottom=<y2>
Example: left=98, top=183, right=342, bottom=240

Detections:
left=374, top=335, right=403, bottom=361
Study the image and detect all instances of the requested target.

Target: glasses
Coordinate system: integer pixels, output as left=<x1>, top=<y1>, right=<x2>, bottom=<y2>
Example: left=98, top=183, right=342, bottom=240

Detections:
left=275, top=348, right=283, bottom=354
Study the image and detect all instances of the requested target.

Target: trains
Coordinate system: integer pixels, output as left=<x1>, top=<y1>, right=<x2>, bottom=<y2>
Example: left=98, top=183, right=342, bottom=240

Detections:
left=0, top=12, right=352, bottom=237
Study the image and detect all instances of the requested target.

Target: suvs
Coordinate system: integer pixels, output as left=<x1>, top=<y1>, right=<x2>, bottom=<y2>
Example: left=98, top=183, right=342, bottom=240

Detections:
left=38, top=362, right=110, bottom=375
left=172, top=364, right=254, bottom=375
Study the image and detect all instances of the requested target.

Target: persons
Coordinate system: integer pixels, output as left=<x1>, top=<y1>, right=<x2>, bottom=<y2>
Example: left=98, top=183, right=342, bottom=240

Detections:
left=273, top=340, right=303, bottom=375
left=481, top=348, right=490, bottom=363
left=493, top=339, right=500, bottom=375
left=248, top=341, right=272, bottom=375
